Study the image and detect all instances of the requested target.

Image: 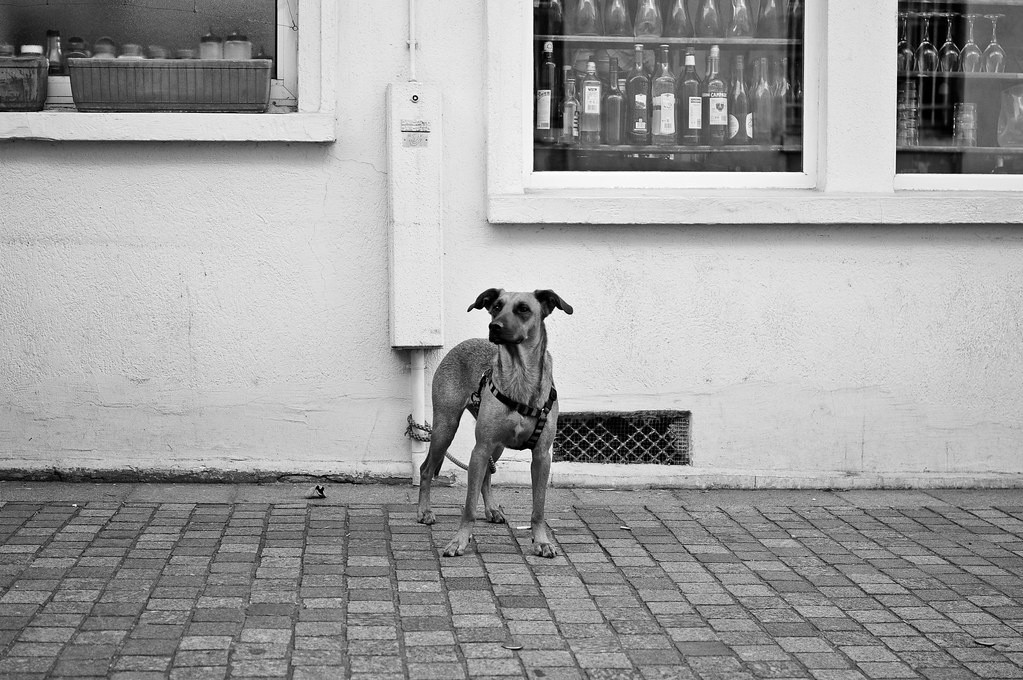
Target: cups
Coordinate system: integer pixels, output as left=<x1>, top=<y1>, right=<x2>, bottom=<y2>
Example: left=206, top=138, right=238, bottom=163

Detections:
left=897, top=79, right=921, bottom=146
left=952, top=103, right=977, bottom=146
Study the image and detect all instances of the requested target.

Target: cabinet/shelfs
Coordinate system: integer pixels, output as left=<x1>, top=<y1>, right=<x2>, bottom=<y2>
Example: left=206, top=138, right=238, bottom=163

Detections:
left=896, top=0, right=1023, bottom=154
left=533, top=33, right=804, bottom=154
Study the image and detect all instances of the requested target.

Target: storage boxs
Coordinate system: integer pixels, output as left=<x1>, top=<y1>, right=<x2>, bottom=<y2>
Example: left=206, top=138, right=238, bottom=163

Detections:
left=0, top=52, right=273, bottom=113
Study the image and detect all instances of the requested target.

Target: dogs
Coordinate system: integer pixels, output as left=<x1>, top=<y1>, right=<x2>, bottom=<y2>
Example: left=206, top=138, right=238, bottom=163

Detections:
left=416, top=288, right=575, bottom=558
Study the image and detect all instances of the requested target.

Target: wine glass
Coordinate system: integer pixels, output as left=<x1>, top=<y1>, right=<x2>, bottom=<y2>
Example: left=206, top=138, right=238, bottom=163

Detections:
left=939, top=12, right=961, bottom=72
left=958, top=13, right=985, bottom=72
left=981, top=14, right=1006, bottom=73
left=898, top=12, right=913, bottom=71
left=914, top=11, right=939, bottom=71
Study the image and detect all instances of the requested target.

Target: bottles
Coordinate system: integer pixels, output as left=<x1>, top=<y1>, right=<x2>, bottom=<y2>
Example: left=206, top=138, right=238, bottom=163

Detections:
left=63, top=37, right=88, bottom=77
left=147, top=48, right=170, bottom=59
left=20, top=44, right=44, bottom=57
left=0, top=45, right=16, bottom=58
left=535, top=41, right=803, bottom=145
left=533, top=0, right=804, bottom=39
left=91, top=45, right=117, bottom=59
left=172, top=50, right=199, bottom=59
left=44, top=30, right=64, bottom=76
left=224, top=32, right=253, bottom=59
left=199, top=27, right=223, bottom=59
left=253, top=45, right=274, bottom=63
left=117, top=44, right=147, bottom=59
